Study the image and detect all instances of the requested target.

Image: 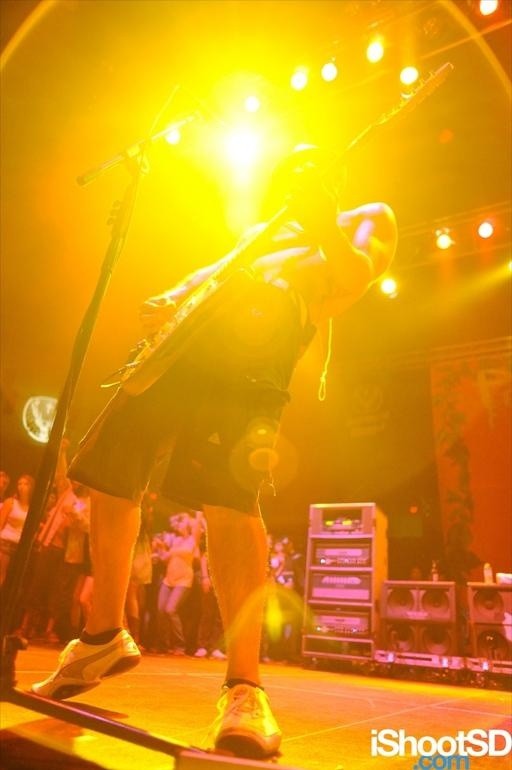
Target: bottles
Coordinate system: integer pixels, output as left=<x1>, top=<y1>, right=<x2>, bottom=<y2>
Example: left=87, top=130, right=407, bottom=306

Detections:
left=431, top=559, right=440, bottom=583
left=483, top=563, right=493, bottom=586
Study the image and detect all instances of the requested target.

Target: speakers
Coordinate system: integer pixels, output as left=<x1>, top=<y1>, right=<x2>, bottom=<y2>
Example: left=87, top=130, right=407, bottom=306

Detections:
left=464, top=580, right=512, bottom=659
left=379, top=580, right=460, bottom=656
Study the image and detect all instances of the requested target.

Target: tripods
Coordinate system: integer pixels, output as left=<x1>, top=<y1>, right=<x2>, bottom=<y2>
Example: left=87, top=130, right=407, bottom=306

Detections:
left=0, top=132, right=281, bottom=770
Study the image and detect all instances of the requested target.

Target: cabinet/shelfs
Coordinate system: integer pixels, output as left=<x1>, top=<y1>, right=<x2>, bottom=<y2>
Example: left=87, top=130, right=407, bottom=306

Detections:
left=300, top=502, right=387, bottom=669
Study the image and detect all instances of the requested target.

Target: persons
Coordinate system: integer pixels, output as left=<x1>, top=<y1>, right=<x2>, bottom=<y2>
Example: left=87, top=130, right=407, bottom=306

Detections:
left=28, top=139, right=399, bottom=758
left=1, top=436, right=303, bottom=668
left=431, top=524, right=482, bottom=582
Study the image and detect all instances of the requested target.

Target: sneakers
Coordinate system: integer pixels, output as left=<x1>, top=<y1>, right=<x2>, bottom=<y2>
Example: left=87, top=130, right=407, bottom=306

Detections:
left=134, top=639, right=228, bottom=659
left=214, top=684, right=282, bottom=757
left=29, top=628, right=143, bottom=702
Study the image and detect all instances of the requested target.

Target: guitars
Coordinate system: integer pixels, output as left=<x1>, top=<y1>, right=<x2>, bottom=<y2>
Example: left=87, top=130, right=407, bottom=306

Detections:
left=119, top=60, right=453, bottom=398
